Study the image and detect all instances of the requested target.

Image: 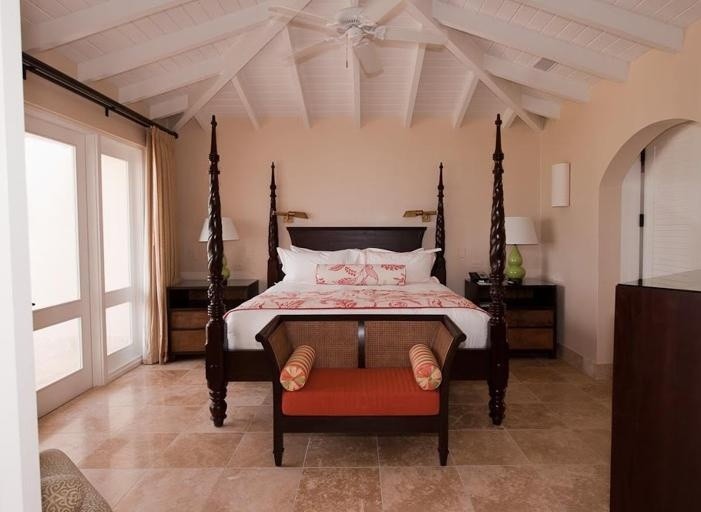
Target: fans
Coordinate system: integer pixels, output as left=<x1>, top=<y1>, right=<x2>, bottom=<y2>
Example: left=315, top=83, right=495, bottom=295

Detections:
left=266, top=0, right=447, bottom=75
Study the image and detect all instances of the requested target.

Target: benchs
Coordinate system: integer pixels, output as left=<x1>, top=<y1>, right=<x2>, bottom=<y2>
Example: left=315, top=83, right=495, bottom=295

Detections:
left=255, top=314, right=467, bottom=466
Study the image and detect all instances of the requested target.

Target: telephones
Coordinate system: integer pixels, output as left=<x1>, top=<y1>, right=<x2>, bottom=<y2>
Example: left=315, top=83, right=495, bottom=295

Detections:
left=469, top=272, right=489, bottom=281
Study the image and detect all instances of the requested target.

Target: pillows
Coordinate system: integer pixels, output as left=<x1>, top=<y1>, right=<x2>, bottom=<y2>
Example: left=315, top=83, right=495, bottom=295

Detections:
left=279, top=345, right=316, bottom=391
left=408, top=343, right=443, bottom=391
left=275, top=245, right=442, bottom=285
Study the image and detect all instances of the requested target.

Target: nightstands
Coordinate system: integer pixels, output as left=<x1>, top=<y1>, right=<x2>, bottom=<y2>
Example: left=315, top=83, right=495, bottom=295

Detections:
left=465, top=279, right=557, bottom=359
left=167, top=278, right=258, bottom=362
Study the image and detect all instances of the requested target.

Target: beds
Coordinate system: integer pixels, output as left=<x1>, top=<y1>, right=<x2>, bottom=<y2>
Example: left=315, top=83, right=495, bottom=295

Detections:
left=204, top=227, right=509, bottom=427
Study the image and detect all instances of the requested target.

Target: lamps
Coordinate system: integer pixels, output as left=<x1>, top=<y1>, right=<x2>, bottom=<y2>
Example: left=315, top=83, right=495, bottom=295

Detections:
left=505, top=217, right=539, bottom=284
left=198, top=217, right=240, bottom=285
left=552, top=163, right=569, bottom=207
